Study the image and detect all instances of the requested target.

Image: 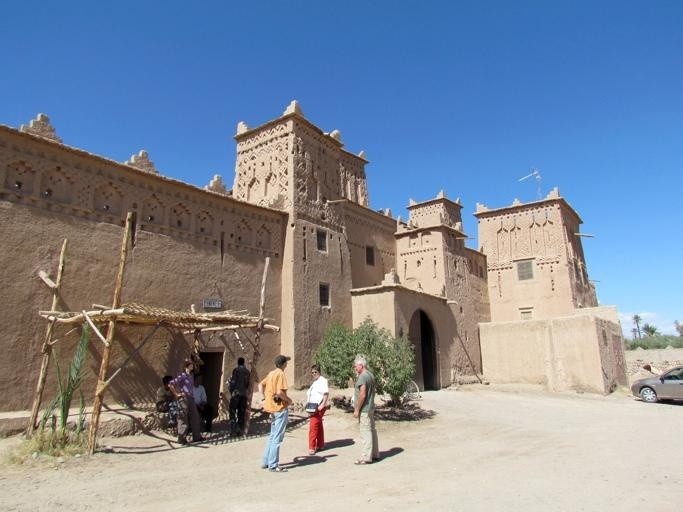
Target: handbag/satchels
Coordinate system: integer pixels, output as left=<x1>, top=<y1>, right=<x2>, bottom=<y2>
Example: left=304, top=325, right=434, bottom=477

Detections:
left=305, top=403, right=318, bottom=413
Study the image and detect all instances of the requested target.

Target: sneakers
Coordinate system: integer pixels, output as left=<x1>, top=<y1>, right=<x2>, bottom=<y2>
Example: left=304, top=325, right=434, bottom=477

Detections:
left=309, top=447, right=324, bottom=455
left=261, top=465, right=287, bottom=473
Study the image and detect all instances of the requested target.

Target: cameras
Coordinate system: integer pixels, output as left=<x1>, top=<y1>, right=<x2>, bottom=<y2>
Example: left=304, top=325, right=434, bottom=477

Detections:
left=273, top=394, right=283, bottom=403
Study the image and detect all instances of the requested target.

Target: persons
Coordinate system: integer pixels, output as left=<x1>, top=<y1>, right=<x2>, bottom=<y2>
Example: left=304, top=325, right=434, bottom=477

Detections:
left=155, top=374, right=177, bottom=423
left=351, top=354, right=382, bottom=465
left=192, top=371, right=214, bottom=434
left=257, top=355, right=294, bottom=472
left=227, top=357, right=252, bottom=438
left=303, top=365, right=329, bottom=456
left=166, top=358, right=206, bottom=443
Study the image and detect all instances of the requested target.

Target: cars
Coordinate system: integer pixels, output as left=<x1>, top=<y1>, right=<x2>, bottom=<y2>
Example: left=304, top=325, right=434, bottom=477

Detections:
left=630, top=365, right=682, bottom=403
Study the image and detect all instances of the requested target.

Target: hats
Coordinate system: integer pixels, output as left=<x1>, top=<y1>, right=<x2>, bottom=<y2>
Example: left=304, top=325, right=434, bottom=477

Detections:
left=275, top=355, right=290, bottom=362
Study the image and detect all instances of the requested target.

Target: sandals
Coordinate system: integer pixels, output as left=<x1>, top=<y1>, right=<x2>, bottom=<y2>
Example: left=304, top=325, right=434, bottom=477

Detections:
left=354, top=458, right=378, bottom=465
